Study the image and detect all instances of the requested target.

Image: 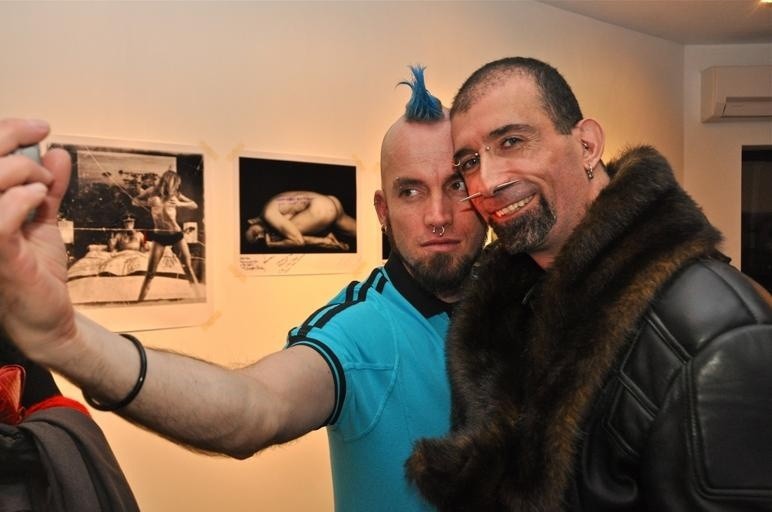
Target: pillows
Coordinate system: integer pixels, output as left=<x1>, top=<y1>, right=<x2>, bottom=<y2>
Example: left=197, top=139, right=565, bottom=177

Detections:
left=146, top=242, right=174, bottom=253
left=89, top=243, right=109, bottom=251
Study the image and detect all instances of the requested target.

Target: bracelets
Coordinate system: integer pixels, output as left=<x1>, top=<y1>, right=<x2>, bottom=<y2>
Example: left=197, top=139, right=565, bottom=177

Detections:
left=82, top=333, right=146, bottom=411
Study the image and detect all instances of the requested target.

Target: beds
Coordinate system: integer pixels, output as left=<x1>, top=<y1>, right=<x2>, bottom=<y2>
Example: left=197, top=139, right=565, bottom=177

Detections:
left=65, top=228, right=195, bottom=304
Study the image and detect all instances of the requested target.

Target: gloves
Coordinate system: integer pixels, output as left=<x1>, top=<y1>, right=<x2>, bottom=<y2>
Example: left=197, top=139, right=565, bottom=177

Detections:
left=0, top=422, right=34, bottom=481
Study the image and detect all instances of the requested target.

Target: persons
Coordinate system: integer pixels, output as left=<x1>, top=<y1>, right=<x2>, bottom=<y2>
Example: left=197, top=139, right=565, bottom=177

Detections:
left=242, top=190, right=356, bottom=252
left=106, top=212, right=149, bottom=253
left=1, top=62, right=490, bottom=512
left=404, top=58, right=772, bottom=511
left=132, top=170, right=199, bottom=301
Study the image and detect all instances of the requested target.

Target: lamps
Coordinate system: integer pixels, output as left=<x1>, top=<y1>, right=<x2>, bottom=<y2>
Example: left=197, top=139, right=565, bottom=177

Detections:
left=183, top=222, right=200, bottom=256
left=58, top=220, right=76, bottom=261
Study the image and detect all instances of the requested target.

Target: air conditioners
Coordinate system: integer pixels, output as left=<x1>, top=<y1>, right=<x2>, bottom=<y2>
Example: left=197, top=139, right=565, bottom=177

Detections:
left=700, top=66, right=772, bottom=123
